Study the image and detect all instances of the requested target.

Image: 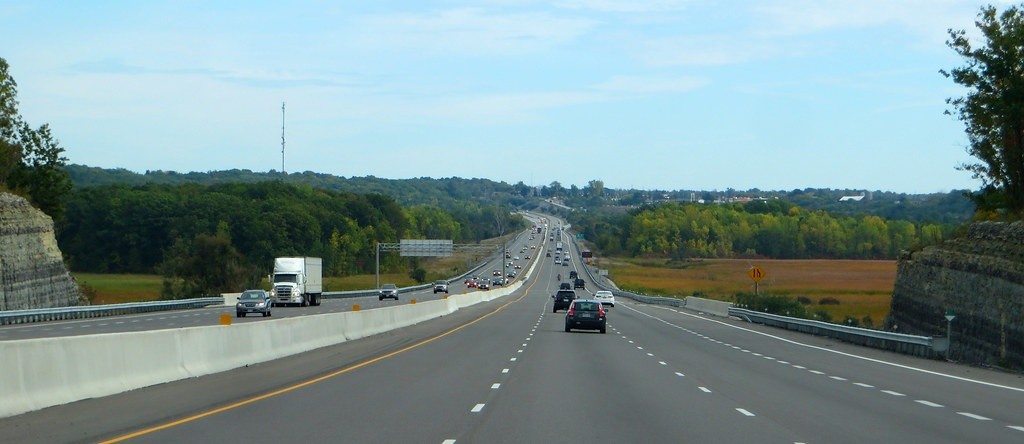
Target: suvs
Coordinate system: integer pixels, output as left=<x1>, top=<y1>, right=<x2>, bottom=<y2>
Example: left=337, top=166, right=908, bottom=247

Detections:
left=236, top=288, right=272, bottom=318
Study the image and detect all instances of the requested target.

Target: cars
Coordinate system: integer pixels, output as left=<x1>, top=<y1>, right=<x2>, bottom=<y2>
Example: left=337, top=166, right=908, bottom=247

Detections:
left=464, top=208, right=587, bottom=292
left=379, top=284, right=398, bottom=301
left=432, top=279, right=450, bottom=294
left=591, top=290, right=615, bottom=307
left=552, top=290, right=580, bottom=314
left=563, top=298, right=609, bottom=335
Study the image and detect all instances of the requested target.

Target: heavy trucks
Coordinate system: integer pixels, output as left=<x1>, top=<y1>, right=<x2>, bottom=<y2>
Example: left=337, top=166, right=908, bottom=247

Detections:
left=267, top=255, right=323, bottom=307
